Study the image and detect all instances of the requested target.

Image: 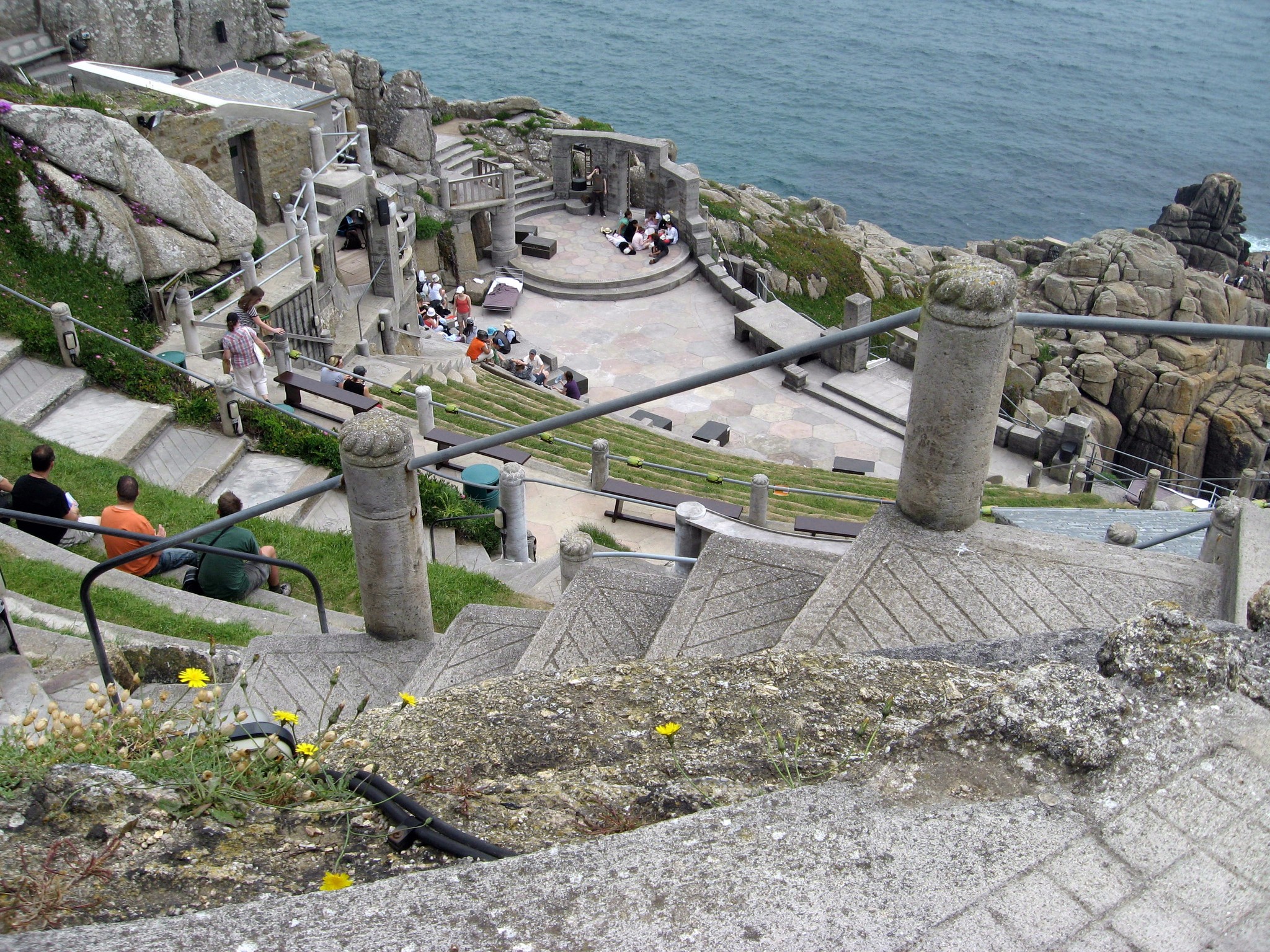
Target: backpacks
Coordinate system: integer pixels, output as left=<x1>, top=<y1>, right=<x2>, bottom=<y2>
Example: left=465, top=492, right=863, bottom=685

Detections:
left=341, top=231, right=362, bottom=250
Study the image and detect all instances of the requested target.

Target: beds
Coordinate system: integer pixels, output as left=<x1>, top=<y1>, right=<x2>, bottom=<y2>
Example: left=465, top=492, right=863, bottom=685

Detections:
left=481, top=266, right=524, bottom=319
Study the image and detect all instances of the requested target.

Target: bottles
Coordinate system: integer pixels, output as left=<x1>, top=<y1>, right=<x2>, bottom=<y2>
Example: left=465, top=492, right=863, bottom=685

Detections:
left=555, top=375, right=563, bottom=384
left=63, top=491, right=79, bottom=508
left=619, top=210, right=622, bottom=220
left=596, top=170, right=598, bottom=174
left=457, top=331, right=460, bottom=337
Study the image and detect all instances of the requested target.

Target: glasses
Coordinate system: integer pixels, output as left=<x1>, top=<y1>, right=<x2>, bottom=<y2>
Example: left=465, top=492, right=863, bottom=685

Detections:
left=630, top=214, right=632, bottom=216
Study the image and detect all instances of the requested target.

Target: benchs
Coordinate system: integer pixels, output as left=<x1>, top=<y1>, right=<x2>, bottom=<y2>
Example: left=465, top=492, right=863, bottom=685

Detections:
left=796, top=516, right=865, bottom=538
left=272, top=370, right=379, bottom=424
left=423, top=425, right=534, bottom=473
left=601, top=478, right=743, bottom=532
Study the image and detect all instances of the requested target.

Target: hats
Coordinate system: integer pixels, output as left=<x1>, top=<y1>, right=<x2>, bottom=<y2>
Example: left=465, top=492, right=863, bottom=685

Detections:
left=603, top=227, right=612, bottom=233
left=432, top=274, right=440, bottom=282
left=426, top=308, right=436, bottom=317
left=624, top=247, right=631, bottom=254
left=644, top=228, right=655, bottom=235
left=500, top=319, right=514, bottom=329
left=530, top=349, right=536, bottom=355
left=487, top=327, right=497, bottom=336
left=456, top=286, right=464, bottom=294
left=663, top=214, right=670, bottom=222
left=416, top=294, right=422, bottom=300
left=477, top=329, right=488, bottom=341
left=328, top=355, right=341, bottom=367
left=354, top=366, right=367, bottom=375
left=466, top=317, right=474, bottom=324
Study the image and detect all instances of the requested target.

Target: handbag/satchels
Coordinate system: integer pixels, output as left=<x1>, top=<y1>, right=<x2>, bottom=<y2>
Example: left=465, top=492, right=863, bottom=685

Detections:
left=244, top=326, right=265, bottom=368
left=181, top=568, right=200, bottom=595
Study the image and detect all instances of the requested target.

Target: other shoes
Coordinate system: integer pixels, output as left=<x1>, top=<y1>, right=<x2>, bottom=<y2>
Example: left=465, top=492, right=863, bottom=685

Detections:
left=649, top=260, right=653, bottom=264
left=612, top=229, right=617, bottom=235
left=587, top=214, right=591, bottom=216
left=266, top=399, right=271, bottom=404
left=602, top=215, right=606, bottom=217
left=602, top=231, right=606, bottom=235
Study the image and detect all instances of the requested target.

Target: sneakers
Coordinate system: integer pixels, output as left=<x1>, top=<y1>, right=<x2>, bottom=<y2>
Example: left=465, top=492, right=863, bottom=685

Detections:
left=280, top=583, right=291, bottom=596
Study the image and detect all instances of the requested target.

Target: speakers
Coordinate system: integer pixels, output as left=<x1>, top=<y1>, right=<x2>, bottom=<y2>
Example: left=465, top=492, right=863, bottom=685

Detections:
left=215, top=21, right=227, bottom=42
left=376, top=196, right=390, bottom=227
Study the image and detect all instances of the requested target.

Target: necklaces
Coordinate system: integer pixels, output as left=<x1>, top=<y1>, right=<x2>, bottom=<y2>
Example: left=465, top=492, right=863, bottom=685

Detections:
left=31, top=470, right=46, bottom=479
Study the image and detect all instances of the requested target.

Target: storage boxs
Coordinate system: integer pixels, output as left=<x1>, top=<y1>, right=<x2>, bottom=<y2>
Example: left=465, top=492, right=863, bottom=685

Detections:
left=631, top=409, right=672, bottom=434
left=692, top=421, right=731, bottom=447
left=515, top=224, right=537, bottom=244
left=521, top=236, right=557, bottom=260
left=559, top=366, right=589, bottom=396
left=832, top=456, right=875, bottom=475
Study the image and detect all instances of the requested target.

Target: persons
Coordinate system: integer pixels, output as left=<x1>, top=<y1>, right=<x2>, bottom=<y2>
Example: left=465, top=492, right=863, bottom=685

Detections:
left=417, top=270, right=580, bottom=401
left=1219, top=250, right=1270, bottom=289
left=191, top=491, right=292, bottom=603
left=0, top=444, right=103, bottom=545
left=100, top=475, right=198, bottom=577
left=587, top=166, right=678, bottom=265
left=222, top=286, right=382, bottom=417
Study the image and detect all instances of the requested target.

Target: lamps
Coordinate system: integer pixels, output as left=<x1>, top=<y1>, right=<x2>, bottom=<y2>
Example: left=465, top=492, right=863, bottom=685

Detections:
left=149, top=706, right=297, bottom=767
left=313, top=314, right=320, bottom=327
left=494, top=505, right=506, bottom=528
left=404, top=322, right=411, bottom=332
left=138, top=110, right=165, bottom=131
left=314, top=244, right=325, bottom=254
left=63, top=331, right=77, bottom=351
left=70, top=35, right=89, bottom=53
left=227, top=399, right=240, bottom=421
left=378, top=320, right=385, bottom=331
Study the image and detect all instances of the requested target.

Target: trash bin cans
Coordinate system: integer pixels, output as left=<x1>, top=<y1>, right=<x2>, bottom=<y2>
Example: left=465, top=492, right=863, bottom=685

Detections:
left=461, top=464, right=500, bottom=512
left=273, top=404, right=294, bottom=414
left=572, top=179, right=587, bottom=191
left=156, top=351, right=187, bottom=370
left=1059, top=441, right=1077, bottom=463
